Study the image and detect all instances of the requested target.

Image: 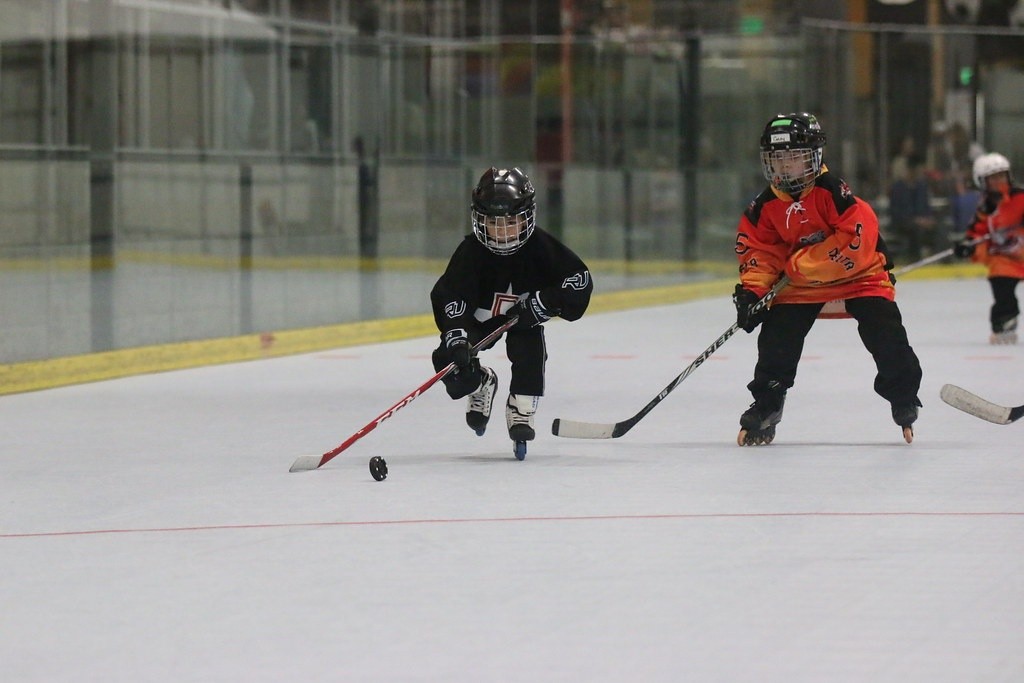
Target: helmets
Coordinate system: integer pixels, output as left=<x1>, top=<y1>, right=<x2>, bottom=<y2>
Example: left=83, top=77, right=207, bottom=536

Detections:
left=760, top=112, right=826, bottom=194
left=469, top=166, right=537, bottom=256
left=973, top=151, right=1011, bottom=186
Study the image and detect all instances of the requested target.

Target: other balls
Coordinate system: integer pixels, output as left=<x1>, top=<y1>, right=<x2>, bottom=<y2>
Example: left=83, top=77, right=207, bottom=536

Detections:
left=369, top=456, right=388, bottom=481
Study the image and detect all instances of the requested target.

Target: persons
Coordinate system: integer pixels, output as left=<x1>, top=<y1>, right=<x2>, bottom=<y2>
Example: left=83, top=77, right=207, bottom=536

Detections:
left=961, top=154, right=1024, bottom=346
left=732, top=112, right=922, bottom=445
left=859, top=125, right=983, bottom=262
left=430, top=168, right=594, bottom=458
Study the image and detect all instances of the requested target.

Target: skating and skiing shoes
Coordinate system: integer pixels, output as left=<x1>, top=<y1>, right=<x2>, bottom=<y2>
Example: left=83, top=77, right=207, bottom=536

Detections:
left=891, top=396, right=922, bottom=442
left=466, top=367, right=498, bottom=436
left=505, top=393, right=535, bottom=460
left=992, top=311, right=1019, bottom=343
left=739, top=378, right=787, bottom=445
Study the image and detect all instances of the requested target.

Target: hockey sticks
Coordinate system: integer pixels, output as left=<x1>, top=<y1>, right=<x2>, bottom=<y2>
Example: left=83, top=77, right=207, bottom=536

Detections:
left=893, top=220, right=1024, bottom=277
left=289, top=315, right=519, bottom=473
left=939, top=384, right=1024, bottom=425
left=551, top=274, right=791, bottom=439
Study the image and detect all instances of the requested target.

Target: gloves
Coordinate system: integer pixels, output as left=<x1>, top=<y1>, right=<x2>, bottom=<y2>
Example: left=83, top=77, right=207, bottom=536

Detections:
left=952, top=236, right=976, bottom=257
left=734, top=284, right=765, bottom=334
left=506, top=291, right=557, bottom=331
left=444, top=340, right=479, bottom=379
